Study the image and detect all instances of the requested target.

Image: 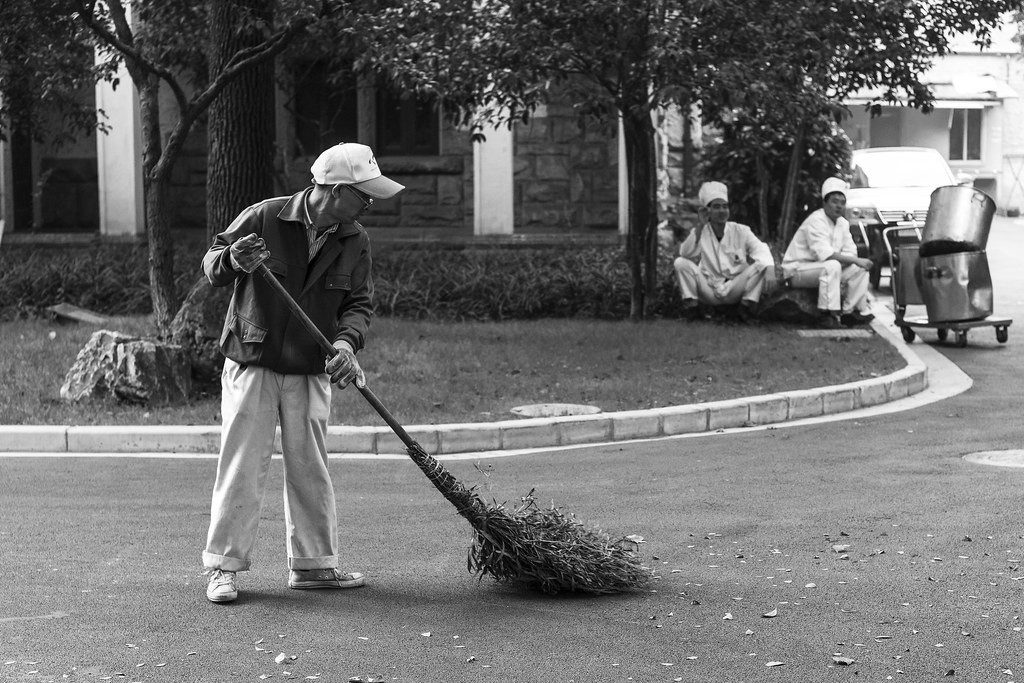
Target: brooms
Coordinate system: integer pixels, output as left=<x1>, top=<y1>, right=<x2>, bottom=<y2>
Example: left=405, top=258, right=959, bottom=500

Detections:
left=254, top=255, right=657, bottom=599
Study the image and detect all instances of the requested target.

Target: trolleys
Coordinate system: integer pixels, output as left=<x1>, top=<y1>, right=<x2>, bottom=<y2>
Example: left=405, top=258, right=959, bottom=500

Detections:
left=883, top=222, right=1012, bottom=344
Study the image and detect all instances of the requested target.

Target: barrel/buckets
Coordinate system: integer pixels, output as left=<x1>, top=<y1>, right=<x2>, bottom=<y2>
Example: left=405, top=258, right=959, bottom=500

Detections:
left=918, top=186, right=996, bottom=258
left=896, top=245, right=923, bottom=306
left=920, top=250, right=993, bottom=323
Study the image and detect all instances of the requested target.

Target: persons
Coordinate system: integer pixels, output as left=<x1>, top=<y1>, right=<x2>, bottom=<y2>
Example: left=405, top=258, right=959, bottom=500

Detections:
left=202, top=142, right=406, bottom=602
left=674, top=181, right=780, bottom=320
left=779, top=177, right=876, bottom=329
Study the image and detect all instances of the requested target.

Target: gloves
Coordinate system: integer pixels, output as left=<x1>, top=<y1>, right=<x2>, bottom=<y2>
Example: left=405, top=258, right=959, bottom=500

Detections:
left=323, top=340, right=367, bottom=390
left=228, top=233, right=272, bottom=274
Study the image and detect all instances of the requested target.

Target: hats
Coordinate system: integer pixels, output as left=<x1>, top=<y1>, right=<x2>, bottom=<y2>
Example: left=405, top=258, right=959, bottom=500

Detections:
left=698, top=181, right=728, bottom=208
left=310, top=142, right=406, bottom=199
left=821, top=176, right=848, bottom=199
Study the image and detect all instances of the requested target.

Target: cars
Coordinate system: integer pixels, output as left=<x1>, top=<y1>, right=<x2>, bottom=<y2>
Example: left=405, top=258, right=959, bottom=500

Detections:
left=843, top=146, right=964, bottom=271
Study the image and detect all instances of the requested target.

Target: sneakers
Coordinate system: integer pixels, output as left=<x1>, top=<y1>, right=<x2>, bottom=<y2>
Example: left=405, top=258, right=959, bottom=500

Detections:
left=288, top=568, right=366, bottom=589
left=200, top=568, right=238, bottom=602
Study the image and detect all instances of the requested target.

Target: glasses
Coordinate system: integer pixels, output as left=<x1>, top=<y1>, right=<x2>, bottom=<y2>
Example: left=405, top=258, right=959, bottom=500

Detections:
left=343, top=185, right=376, bottom=213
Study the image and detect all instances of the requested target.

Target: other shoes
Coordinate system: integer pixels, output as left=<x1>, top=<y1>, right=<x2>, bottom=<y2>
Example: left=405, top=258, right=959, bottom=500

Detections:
left=739, top=310, right=754, bottom=323
left=815, top=315, right=848, bottom=329
left=683, top=305, right=699, bottom=322
left=840, top=309, right=875, bottom=325
